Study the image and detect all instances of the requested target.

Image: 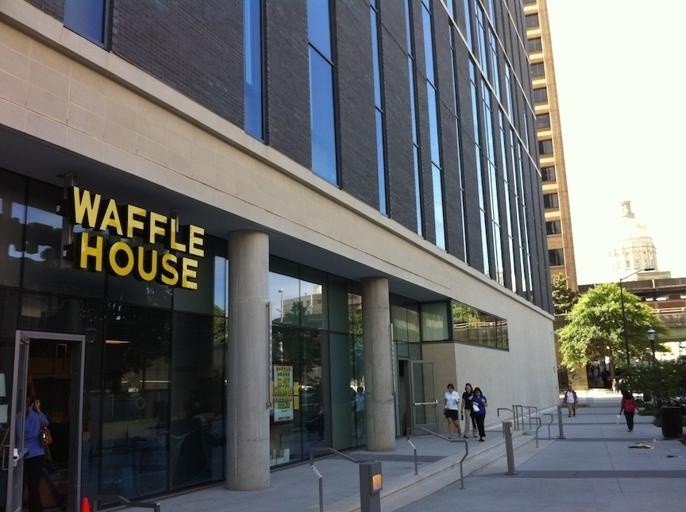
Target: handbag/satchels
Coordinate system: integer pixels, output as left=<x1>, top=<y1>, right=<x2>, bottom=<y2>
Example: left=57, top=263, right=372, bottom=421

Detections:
left=40, top=426, right=53, bottom=448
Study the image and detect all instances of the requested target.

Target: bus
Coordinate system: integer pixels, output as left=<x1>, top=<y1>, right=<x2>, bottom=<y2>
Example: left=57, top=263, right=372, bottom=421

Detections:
left=82, top=379, right=223, bottom=446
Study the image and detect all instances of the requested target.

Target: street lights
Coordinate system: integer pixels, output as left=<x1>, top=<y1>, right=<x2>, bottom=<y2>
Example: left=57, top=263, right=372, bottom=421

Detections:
left=618, top=265, right=656, bottom=398
left=646, top=324, right=662, bottom=409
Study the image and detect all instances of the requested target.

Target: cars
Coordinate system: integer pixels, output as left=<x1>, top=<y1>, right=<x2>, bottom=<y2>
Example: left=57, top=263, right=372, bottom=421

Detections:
left=637, top=389, right=686, bottom=409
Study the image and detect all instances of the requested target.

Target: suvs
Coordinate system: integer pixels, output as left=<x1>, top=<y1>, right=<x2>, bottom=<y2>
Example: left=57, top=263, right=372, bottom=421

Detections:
left=292, top=384, right=355, bottom=428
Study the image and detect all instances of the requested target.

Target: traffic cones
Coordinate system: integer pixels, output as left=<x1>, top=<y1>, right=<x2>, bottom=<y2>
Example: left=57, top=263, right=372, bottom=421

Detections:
left=80, top=498, right=90, bottom=512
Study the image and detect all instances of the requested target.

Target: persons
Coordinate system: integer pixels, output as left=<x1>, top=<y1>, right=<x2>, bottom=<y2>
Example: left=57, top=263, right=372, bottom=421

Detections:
left=443, top=383, right=462, bottom=439
left=460, top=383, right=480, bottom=438
left=355, top=387, right=365, bottom=425
left=563, top=385, right=577, bottom=417
left=468, top=388, right=487, bottom=442
left=619, top=391, right=640, bottom=432
left=15, top=396, right=51, bottom=512
